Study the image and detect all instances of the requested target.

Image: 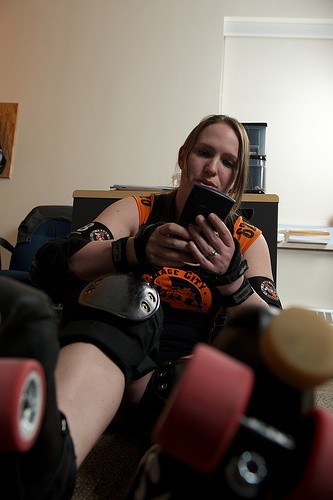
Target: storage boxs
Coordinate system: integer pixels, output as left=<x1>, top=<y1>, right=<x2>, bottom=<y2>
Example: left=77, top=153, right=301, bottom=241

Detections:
left=240, top=122, right=268, bottom=193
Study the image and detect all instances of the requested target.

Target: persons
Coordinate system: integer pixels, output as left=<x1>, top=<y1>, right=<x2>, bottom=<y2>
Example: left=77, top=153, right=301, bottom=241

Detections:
left=0, top=115, right=333, bottom=500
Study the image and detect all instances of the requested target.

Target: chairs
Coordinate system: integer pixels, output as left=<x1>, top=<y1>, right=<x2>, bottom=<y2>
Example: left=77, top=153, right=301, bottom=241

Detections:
left=0, top=205, right=333, bottom=500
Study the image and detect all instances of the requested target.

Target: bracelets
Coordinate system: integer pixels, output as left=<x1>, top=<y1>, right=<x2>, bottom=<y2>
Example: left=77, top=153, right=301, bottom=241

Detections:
left=112, top=237, right=129, bottom=272
left=221, top=276, right=254, bottom=307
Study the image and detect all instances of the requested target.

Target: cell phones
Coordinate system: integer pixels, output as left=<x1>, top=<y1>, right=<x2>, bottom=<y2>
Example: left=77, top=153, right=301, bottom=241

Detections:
left=168, top=184, right=237, bottom=263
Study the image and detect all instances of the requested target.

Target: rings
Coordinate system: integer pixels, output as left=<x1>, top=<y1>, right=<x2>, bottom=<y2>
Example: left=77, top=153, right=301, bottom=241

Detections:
left=208, top=250, right=216, bottom=258
